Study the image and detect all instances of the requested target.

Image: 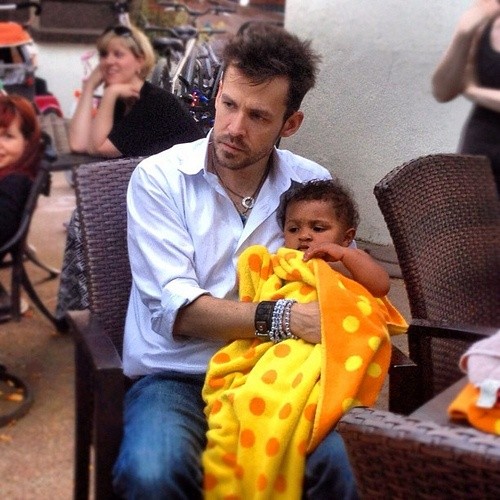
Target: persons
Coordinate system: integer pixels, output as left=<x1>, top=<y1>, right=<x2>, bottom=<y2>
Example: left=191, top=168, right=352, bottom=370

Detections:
left=0, top=93, right=42, bottom=264
left=276, top=178, right=392, bottom=297
left=430, top=0, right=500, bottom=202
left=55, top=22, right=206, bottom=320
left=109, top=24, right=360, bottom=500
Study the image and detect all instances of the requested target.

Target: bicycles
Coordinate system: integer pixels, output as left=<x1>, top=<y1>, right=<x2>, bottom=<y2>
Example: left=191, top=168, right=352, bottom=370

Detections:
left=113, top=0, right=238, bottom=133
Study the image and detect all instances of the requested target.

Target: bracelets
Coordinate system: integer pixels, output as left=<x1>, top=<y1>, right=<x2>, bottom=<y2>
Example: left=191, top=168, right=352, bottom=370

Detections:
left=254, top=298, right=300, bottom=344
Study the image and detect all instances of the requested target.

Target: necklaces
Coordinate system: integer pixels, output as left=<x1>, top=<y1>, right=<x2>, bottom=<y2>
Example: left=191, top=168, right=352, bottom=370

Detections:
left=211, top=144, right=273, bottom=213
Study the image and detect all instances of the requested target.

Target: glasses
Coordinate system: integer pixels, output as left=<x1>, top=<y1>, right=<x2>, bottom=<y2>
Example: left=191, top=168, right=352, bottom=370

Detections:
left=104, top=25, right=143, bottom=53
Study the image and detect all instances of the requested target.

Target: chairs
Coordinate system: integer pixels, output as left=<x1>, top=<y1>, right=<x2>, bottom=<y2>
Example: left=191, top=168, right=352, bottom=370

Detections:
left=66, top=155, right=420, bottom=500
left=373, top=154, right=500, bottom=409
left=0, top=151, right=73, bottom=338
left=335, top=405, right=500, bottom=500
